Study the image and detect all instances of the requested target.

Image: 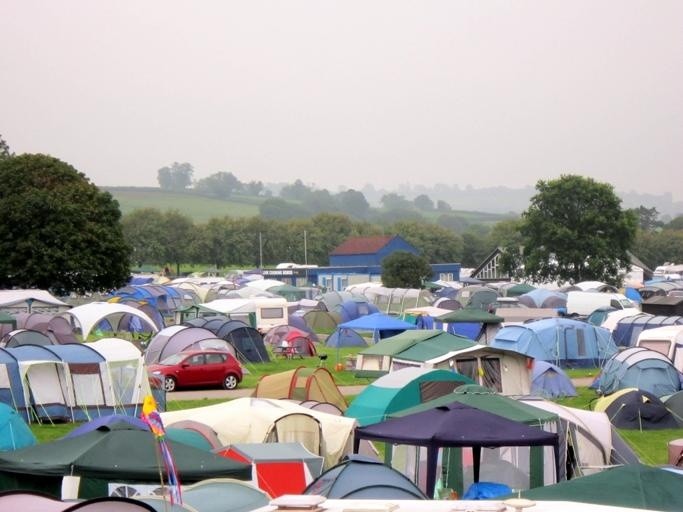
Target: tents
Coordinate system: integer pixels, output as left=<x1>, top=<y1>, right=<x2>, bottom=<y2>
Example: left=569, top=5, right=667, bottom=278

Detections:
left=0, top=264, right=683, bottom=511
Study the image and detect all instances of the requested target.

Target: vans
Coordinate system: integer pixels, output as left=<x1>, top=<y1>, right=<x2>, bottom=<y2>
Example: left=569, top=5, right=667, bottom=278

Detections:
left=654, top=265, right=683, bottom=274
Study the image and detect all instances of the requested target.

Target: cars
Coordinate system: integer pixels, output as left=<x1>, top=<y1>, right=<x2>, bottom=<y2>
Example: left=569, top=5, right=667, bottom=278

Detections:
left=145, top=350, right=243, bottom=391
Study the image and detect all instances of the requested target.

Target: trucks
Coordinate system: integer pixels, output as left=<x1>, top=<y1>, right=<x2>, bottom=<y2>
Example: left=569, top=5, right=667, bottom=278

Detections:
left=566, top=291, right=637, bottom=321
left=276, top=263, right=318, bottom=269
